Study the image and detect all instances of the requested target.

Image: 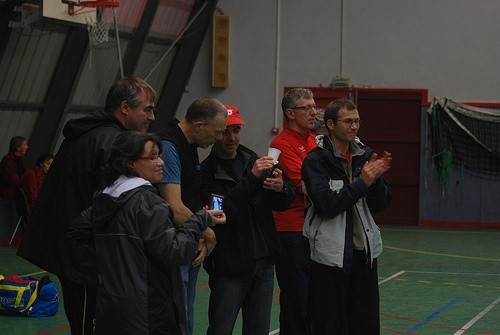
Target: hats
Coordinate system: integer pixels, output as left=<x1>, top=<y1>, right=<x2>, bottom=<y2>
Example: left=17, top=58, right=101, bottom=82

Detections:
left=224, top=104, right=246, bottom=126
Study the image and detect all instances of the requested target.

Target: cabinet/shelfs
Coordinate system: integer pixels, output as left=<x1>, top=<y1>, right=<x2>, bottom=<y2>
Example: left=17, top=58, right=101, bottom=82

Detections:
left=284, top=86, right=428, bottom=227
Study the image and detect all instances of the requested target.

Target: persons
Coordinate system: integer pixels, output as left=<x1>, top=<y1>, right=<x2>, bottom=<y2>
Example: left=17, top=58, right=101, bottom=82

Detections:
left=302, top=98, right=394, bottom=335
left=0, top=136, right=32, bottom=235
left=16, top=75, right=329, bottom=335
left=27, top=152, right=54, bottom=202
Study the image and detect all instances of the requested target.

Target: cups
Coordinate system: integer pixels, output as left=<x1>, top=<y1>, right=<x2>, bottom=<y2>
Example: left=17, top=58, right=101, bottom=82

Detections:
left=268, top=148, right=281, bottom=164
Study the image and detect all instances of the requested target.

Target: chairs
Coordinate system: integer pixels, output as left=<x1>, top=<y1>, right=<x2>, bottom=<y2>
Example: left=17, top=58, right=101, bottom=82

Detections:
left=6, top=183, right=32, bottom=246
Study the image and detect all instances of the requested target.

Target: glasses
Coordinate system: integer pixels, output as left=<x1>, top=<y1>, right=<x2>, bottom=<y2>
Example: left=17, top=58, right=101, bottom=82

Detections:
left=291, top=105, right=316, bottom=111
left=335, top=119, right=359, bottom=125
left=133, top=154, right=162, bottom=161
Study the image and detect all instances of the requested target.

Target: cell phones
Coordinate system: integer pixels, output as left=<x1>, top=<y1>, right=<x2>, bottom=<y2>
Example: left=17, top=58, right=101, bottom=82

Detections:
left=266, top=161, right=279, bottom=178
left=209, top=193, right=224, bottom=218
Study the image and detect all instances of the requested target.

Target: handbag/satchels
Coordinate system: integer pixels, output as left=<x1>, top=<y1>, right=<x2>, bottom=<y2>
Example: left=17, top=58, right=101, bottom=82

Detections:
left=0, top=275, right=58, bottom=317
